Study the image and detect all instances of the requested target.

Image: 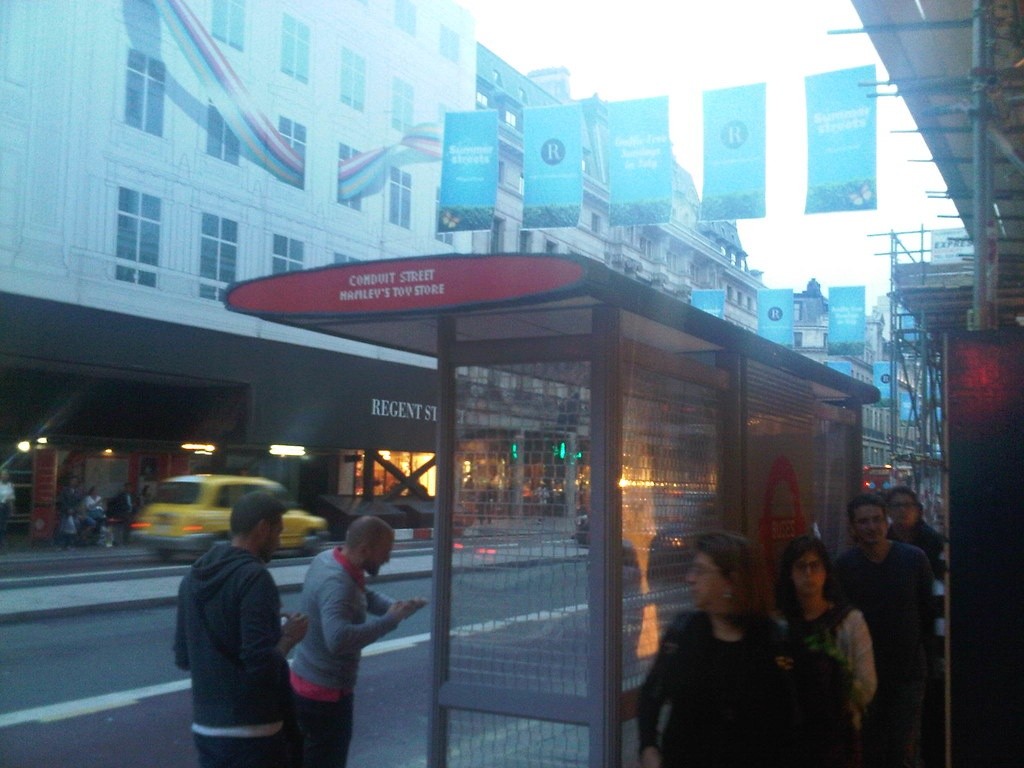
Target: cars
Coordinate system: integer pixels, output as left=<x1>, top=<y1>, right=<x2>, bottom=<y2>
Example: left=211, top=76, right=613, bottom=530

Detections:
left=574, top=490, right=720, bottom=574
left=131, top=474, right=326, bottom=562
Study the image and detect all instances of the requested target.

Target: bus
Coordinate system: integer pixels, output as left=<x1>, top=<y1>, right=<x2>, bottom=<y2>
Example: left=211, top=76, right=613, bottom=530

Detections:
left=863, top=465, right=913, bottom=496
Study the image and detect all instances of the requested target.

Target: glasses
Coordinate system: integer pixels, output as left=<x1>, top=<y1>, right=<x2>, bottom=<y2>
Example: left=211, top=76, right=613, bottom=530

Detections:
left=886, top=499, right=919, bottom=511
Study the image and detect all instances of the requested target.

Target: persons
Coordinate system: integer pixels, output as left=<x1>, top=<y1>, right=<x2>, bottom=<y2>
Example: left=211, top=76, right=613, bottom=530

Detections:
left=0, top=470, right=15, bottom=554
left=466, top=479, right=562, bottom=525
left=637, top=532, right=832, bottom=768
left=286, top=514, right=427, bottom=768
left=885, top=486, right=944, bottom=675
left=823, top=493, right=936, bottom=768
left=175, top=492, right=310, bottom=768
left=764, top=536, right=876, bottom=768
left=57, top=477, right=152, bottom=546
